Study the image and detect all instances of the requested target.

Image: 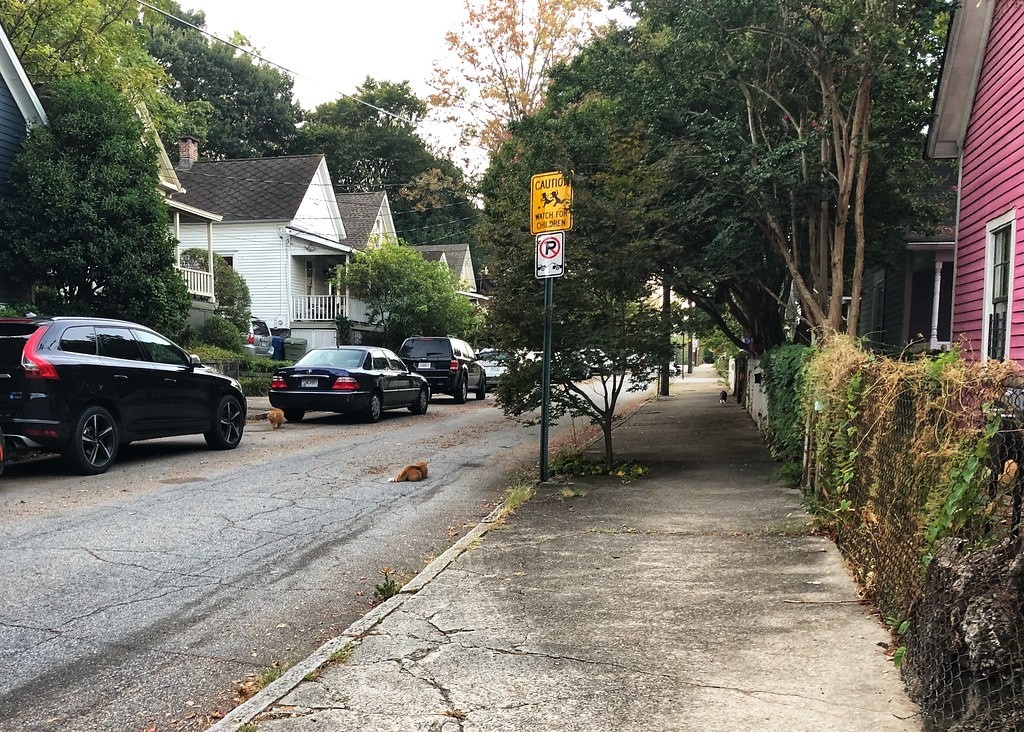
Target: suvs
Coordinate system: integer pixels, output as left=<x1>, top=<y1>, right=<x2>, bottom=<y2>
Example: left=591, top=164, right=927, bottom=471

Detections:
left=475, top=347, right=544, bottom=393
left=246, top=316, right=274, bottom=358
left=1, top=312, right=249, bottom=476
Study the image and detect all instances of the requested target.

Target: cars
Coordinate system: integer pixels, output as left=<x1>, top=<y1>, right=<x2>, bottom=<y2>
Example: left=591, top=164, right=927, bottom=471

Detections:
left=398, top=335, right=487, bottom=403
left=270, top=341, right=430, bottom=425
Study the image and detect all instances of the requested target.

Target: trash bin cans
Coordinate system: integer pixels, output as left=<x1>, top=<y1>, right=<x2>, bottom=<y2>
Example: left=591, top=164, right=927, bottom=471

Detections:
left=282, top=338, right=307, bottom=362
left=272, top=336, right=285, bottom=361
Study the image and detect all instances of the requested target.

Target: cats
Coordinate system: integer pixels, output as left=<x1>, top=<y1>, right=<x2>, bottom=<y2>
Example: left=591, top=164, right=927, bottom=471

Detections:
left=388, top=461, right=428, bottom=482
left=268, top=408, right=288, bottom=430
